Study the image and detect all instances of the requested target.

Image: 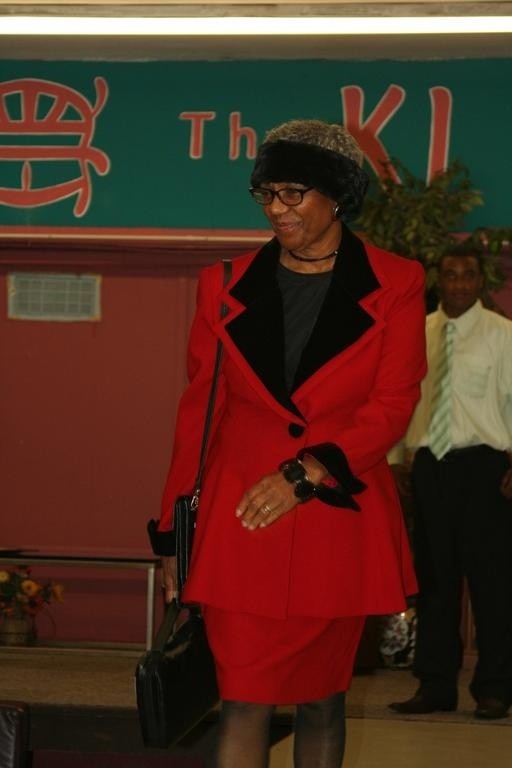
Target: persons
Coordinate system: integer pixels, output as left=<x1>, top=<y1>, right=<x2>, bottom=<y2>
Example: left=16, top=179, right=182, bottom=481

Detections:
left=389, top=242, right=509, bottom=720
left=144, top=118, right=430, bottom=766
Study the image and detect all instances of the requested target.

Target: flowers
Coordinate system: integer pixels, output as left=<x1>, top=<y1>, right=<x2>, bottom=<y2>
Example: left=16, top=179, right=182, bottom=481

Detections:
left=1, top=563, right=67, bottom=621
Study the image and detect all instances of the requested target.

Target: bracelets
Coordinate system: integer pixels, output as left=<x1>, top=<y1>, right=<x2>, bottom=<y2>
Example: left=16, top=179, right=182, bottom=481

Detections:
left=273, top=451, right=322, bottom=511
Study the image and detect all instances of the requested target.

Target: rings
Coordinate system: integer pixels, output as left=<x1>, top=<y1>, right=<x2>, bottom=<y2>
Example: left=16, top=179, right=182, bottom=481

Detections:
left=259, top=503, right=272, bottom=517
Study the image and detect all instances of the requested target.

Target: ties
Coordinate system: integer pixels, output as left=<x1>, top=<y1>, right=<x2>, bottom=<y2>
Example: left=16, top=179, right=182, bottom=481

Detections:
left=428, top=323, right=454, bottom=460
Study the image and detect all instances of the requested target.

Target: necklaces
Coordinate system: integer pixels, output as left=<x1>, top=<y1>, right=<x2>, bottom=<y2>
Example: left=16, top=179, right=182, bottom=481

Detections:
left=278, top=247, right=340, bottom=264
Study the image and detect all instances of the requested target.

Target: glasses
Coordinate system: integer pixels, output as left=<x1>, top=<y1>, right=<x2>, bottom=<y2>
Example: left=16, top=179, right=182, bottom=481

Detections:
left=251, top=186, right=313, bottom=206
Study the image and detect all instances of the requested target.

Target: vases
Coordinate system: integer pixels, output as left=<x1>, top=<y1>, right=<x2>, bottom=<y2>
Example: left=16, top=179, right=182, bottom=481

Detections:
left=376, top=594, right=420, bottom=672
left=1, top=615, right=38, bottom=647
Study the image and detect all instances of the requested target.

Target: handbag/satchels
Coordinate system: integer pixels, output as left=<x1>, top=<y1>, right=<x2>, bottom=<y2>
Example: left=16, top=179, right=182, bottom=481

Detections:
left=174, top=496, right=198, bottom=610
left=136, top=617, right=218, bottom=746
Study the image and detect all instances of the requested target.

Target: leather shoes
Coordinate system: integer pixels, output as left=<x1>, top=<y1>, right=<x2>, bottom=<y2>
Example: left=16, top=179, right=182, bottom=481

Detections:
left=389, top=689, right=457, bottom=713
left=475, top=695, right=507, bottom=718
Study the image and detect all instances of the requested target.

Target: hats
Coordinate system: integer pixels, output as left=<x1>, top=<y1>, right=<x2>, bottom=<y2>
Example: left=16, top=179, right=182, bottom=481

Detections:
left=250, top=119, right=365, bottom=221
